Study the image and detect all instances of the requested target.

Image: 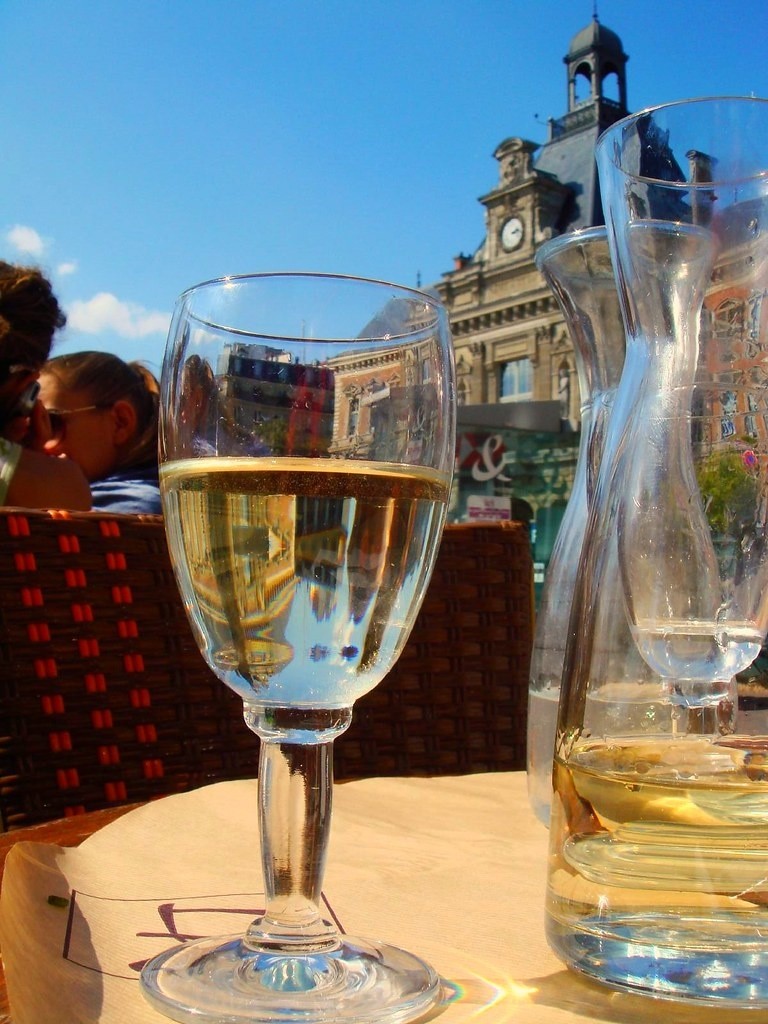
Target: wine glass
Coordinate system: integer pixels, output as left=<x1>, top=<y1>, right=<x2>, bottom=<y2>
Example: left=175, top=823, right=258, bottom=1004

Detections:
left=619, top=384, right=768, bottom=777
left=138, top=272, right=457, bottom=1024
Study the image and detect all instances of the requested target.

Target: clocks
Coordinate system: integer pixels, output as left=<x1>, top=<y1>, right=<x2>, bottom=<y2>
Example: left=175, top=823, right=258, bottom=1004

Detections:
left=500, top=216, right=524, bottom=253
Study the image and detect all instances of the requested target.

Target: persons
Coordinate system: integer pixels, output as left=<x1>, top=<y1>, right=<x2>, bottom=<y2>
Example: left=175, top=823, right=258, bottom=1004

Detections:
left=40, top=350, right=162, bottom=517
left=0, top=260, right=93, bottom=513
left=177, top=353, right=269, bottom=457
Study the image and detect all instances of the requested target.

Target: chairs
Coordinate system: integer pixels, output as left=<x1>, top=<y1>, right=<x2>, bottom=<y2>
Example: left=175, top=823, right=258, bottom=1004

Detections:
left=0, top=504, right=538, bottom=833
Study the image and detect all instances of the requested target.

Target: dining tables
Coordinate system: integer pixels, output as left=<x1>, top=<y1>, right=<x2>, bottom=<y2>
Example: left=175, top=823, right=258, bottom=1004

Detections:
left=0, top=783, right=767, bottom=1024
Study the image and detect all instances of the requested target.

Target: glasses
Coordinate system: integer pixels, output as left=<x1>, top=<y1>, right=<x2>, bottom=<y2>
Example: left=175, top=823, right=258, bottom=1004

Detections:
left=46, top=405, right=96, bottom=431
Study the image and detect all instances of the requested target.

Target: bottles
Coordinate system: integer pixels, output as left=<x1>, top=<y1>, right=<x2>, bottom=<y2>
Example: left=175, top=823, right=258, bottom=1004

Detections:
left=544, top=95, right=768, bottom=1007
left=526, top=219, right=768, bottom=833
